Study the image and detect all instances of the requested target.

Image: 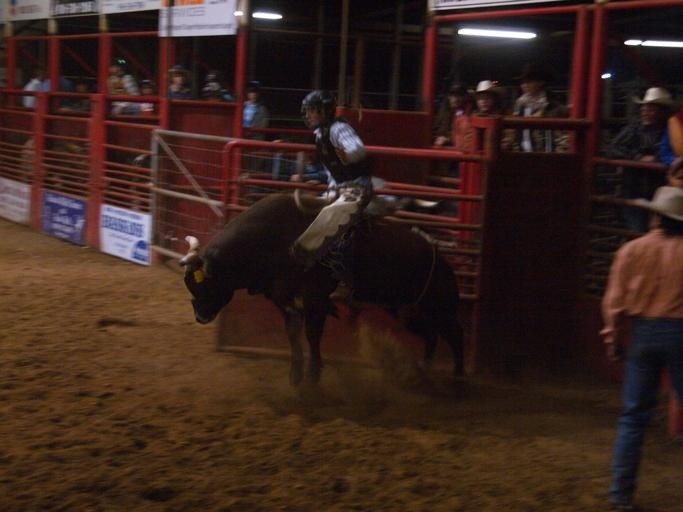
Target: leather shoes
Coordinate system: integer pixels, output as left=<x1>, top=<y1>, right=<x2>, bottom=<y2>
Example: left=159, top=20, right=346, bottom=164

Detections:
left=326, top=278, right=355, bottom=300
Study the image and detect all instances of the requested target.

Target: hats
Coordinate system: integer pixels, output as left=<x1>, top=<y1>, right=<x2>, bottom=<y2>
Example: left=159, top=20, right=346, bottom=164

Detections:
left=508, top=63, right=555, bottom=86
left=105, top=58, right=261, bottom=93
left=625, top=186, right=682, bottom=222
left=629, top=86, right=680, bottom=107
left=447, top=81, right=464, bottom=95
left=466, top=79, right=511, bottom=98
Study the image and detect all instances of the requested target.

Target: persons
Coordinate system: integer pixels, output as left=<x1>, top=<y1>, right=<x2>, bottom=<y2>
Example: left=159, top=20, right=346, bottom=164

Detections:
left=670, top=153, right=682, bottom=184
left=602, top=184, right=682, bottom=509
left=285, top=88, right=374, bottom=302
left=23, top=54, right=272, bottom=141
left=604, top=84, right=674, bottom=231
left=429, top=74, right=617, bottom=160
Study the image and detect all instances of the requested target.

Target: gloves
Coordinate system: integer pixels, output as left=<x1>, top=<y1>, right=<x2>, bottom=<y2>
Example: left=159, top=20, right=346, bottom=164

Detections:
left=605, top=345, right=623, bottom=379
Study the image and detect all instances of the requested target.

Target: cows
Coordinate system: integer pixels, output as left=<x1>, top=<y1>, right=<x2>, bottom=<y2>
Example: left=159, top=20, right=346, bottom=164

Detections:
left=175, top=191, right=466, bottom=395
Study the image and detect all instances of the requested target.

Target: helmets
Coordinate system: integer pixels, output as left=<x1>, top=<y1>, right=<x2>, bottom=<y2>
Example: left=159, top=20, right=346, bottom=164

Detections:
left=301, top=88, right=337, bottom=129
